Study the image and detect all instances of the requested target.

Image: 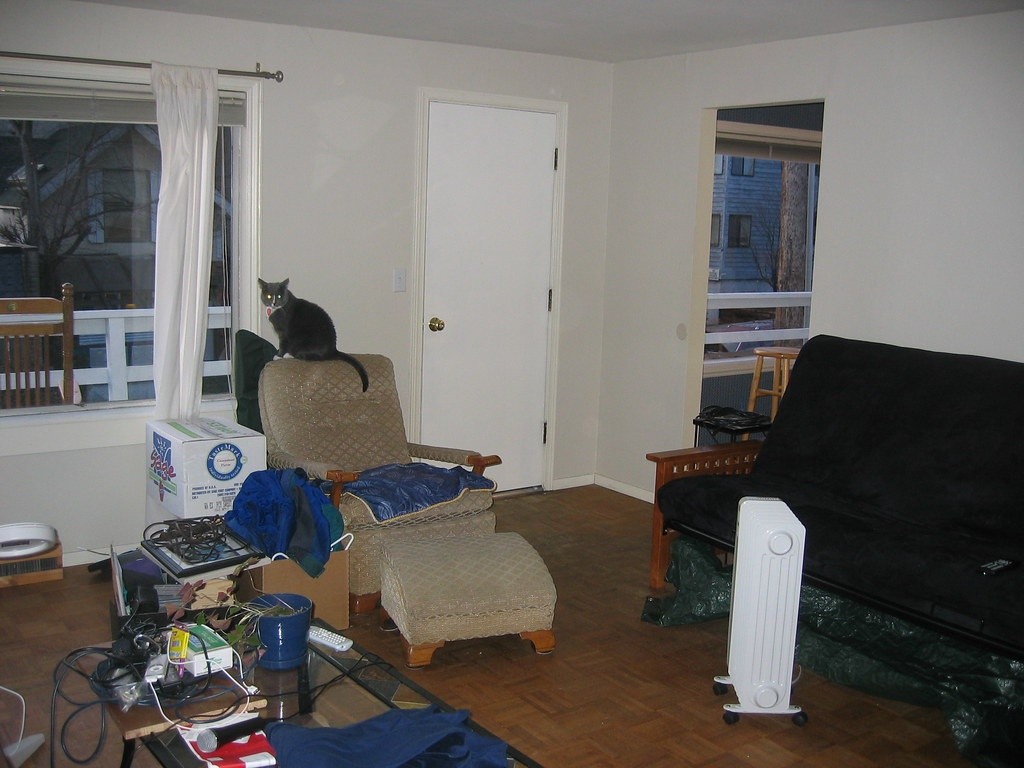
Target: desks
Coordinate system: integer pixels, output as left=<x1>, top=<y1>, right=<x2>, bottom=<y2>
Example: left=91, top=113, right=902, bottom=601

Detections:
left=82, top=617, right=561, bottom=768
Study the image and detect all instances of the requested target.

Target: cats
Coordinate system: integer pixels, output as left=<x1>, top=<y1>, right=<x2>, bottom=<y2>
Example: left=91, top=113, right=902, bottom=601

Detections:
left=257, top=278, right=369, bottom=393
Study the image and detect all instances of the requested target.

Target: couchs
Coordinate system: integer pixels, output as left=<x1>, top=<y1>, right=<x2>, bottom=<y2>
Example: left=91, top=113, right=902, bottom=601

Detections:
left=252, top=356, right=509, bottom=621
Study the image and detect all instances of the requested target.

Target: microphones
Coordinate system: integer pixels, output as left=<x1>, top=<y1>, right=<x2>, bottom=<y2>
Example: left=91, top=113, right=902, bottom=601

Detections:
left=197, top=717, right=280, bottom=753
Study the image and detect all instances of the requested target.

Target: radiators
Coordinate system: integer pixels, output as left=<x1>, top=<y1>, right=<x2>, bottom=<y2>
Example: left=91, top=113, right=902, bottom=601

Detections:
left=722, top=496, right=808, bottom=710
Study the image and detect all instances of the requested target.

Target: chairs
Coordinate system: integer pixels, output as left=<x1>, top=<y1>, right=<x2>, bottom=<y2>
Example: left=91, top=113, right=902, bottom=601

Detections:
left=0, top=281, right=75, bottom=410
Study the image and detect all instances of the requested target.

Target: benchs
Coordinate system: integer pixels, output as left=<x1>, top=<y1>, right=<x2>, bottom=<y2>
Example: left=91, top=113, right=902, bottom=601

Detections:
left=629, top=335, right=1024, bottom=670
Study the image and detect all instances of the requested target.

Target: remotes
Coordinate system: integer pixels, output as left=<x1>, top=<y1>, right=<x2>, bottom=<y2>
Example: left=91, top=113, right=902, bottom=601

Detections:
left=309, top=626, right=353, bottom=652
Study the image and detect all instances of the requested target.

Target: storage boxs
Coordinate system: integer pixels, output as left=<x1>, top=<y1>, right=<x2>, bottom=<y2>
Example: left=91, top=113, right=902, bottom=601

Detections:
left=0, top=536, right=64, bottom=590
left=143, top=418, right=266, bottom=520
left=167, top=623, right=233, bottom=677
left=145, top=495, right=180, bottom=539
left=238, top=545, right=353, bottom=636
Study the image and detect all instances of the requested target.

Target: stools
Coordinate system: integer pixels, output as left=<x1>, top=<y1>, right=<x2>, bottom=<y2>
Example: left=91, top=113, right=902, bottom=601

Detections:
left=373, top=529, right=561, bottom=668
left=743, top=342, right=804, bottom=444
